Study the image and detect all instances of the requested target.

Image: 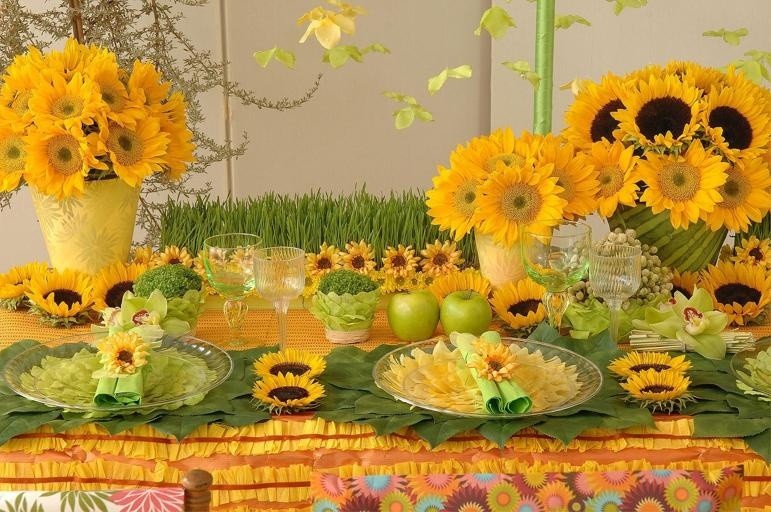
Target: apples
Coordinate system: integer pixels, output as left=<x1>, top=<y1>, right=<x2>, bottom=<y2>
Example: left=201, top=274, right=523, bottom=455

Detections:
left=441, top=289, right=492, bottom=337
left=388, top=289, right=440, bottom=341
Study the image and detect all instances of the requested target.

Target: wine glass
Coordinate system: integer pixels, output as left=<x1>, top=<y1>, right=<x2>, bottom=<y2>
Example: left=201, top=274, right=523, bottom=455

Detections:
left=203, top=231, right=307, bottom=348
left=521, top=217, right=641, bottom=353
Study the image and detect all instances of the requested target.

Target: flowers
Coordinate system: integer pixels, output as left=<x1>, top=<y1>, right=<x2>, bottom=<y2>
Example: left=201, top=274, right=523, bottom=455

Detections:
left=0, top=38, right=771, bottom=512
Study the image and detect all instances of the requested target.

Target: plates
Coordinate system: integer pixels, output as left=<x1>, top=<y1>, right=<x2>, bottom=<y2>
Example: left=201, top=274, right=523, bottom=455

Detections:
left=371, top=336, right=605, bottom=421
left=2, top=332, right=234, bottom=412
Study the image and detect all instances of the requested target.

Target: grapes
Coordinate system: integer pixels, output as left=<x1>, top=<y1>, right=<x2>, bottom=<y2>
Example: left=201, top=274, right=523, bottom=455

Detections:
left=567, top=226, right=673, bottom=309
